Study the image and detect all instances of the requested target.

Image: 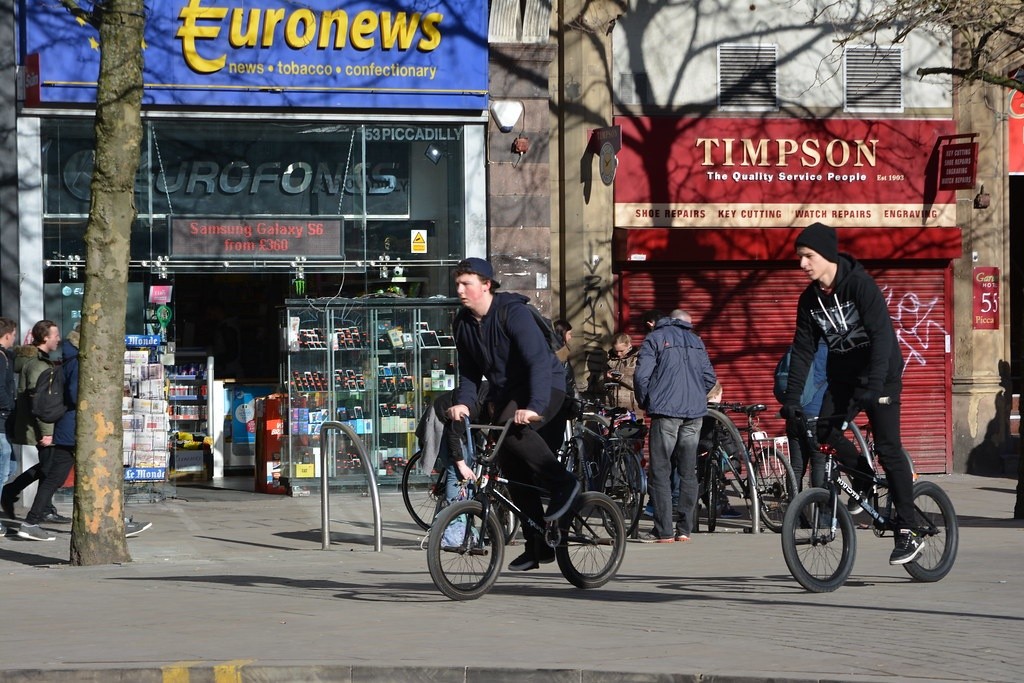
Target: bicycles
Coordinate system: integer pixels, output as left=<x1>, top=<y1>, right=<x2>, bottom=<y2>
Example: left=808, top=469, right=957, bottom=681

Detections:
left=773, top=402, right=961, bottom=594
left=399, top=380, right=920, bottom=545
left=428, top=416, right=628, bottom=603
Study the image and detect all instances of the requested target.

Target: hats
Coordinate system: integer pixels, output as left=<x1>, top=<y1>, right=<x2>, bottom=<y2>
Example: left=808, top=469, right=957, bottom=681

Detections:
left=458, top=258, right=501, bottom=289
left=795, top=223, right=838, bottom=264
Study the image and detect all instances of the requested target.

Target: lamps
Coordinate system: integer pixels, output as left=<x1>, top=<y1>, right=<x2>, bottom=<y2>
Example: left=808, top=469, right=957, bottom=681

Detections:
left=424, top=142, right=452, bottom=164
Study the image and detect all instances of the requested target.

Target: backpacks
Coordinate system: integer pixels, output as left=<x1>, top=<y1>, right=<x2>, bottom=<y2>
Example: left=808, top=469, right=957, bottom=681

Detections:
left=29, top=355, right=76, bottom=424
left=499, top=302, right=566, bottom=353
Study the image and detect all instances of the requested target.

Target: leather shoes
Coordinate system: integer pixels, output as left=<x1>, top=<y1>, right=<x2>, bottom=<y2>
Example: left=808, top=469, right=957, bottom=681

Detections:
left=1, top=497, right=20, bottom=519
left=39, top=513, right=71, bottom=524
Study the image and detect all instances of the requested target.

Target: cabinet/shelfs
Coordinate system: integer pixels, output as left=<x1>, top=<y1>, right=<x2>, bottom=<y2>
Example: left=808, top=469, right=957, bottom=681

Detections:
left=282, top=303, right=460, bottom=490
left=165, top=352, right=215, bottom=482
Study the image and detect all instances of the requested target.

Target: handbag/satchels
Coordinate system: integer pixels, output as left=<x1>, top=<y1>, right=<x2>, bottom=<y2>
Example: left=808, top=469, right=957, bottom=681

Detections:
left=774, top=344, right=815, bottom=408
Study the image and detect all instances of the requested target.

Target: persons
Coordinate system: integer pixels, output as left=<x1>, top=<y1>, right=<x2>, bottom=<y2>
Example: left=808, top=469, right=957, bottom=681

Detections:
left=773, top=335, right=831, bottom=527
left=0, top=317, right=17, bottom=536
left=206, top=299, right=241, bottom=373
left=16, top=325, right=152, bottom=542
left=631, top=309, right=741, bottom=544
left=1, top=320, right=72, bottom=523
left=415, top=257, right=579, bottom=571
left=597, top=332, right=645, bottom=503
left=781, top=223, right=925, bottom=565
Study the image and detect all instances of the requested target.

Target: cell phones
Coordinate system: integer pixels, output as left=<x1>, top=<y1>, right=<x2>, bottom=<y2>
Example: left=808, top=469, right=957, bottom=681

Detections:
left=611, top=372, right=621, bottom=377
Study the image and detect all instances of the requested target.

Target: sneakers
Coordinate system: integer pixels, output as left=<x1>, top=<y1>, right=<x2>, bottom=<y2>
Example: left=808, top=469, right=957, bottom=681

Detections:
left=889, top=529, right=926, bottom=565
left=639, top=532, right=675, bottom=543
left=721, top=508, right=743, bottom=518
left=124, top=515, right=152, bottom=537
left=675, top=534, right=690, bottom=541
left=508, top=539, right=555, bottom=571
left=0, top=522, right=19, bottom=537
left=543, top=470, right=581, bottom=522
left=847, top=478, right=875, bottom=514
left=16, top=523, right=56, bottom=541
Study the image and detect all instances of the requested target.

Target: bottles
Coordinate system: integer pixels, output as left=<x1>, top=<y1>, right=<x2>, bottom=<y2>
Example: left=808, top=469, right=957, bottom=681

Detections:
left=336, top=451, right=362, bottom=474
left=337, top=328, right=370, bottom=349
left=341, top=408, right=362, bottom=422
left=448, top=363, right=454, bottom=374
left=169, top=363, right=207, bottom=380
left=298, top=330, right=326, bottom=348
left=290, top=371, right=328, bottom=410
left=433, top=360, right=439, bottom=370
left=382, top=439, right=393, bottom=450
left=383, top=405, right=398, bottom=417
left=335, top=370, right=365, bottom=391
left=170, top=383, right=207, bottom=395
left=379, top=366, right=415, bottom=403
left=400, top=406, right=414, bottom=418
left=304, top=453, right=310, bottom=464
left=386, top=457, right=415, bottom=476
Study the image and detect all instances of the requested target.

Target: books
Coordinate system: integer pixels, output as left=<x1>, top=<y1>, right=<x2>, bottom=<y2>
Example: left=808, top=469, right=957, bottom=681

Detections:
left=122, top=351, right=170, bottom=468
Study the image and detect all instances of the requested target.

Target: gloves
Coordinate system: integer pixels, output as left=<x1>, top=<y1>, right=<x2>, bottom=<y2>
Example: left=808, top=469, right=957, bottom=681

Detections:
left=779, top=399, right=809, bottom=436
left=854, top=379, right=884, bottom=412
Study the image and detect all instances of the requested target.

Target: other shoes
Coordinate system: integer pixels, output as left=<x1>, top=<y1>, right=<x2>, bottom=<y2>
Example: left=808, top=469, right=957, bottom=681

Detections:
left=768, top=510, right=785, bottom=523
left=641, top=514, right=653, bottom=520
left=809, top=521, right=831, bottom=529
